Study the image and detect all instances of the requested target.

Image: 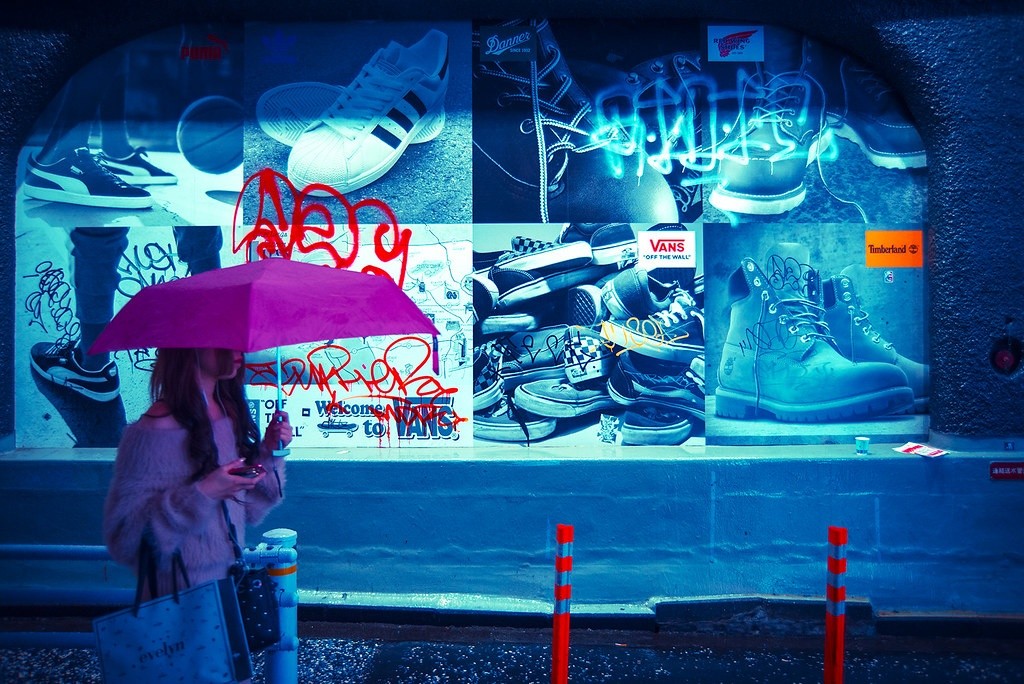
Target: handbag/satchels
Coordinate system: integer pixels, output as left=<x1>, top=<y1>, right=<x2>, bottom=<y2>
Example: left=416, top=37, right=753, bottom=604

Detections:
left=91, top=527, right=255, bottom=684
left=218, top=499, right=282, bottom=650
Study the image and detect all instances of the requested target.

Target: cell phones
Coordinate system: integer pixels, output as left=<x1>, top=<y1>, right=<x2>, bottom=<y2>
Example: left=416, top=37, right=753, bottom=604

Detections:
left=228, top=464, right=267, bottom=478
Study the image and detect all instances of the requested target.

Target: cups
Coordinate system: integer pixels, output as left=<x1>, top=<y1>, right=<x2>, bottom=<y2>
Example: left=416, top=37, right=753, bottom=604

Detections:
left=854, top=437, right=870, bottom=455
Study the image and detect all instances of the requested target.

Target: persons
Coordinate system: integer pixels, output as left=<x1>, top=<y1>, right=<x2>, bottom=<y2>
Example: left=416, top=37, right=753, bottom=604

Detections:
left=30, top=228, right=227, bottom=403
left=26, top=43, right=177, bottom=209
left=104, top=350, right=292, bottom=684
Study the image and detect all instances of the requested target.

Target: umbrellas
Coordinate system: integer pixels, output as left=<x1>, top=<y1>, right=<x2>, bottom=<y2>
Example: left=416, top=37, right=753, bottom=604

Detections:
left=87, top=258, right=439, bottom=455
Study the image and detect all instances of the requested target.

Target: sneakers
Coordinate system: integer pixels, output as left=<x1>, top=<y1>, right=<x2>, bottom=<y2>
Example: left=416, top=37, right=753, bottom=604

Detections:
left=27, top=29, right=936, bottom=446
